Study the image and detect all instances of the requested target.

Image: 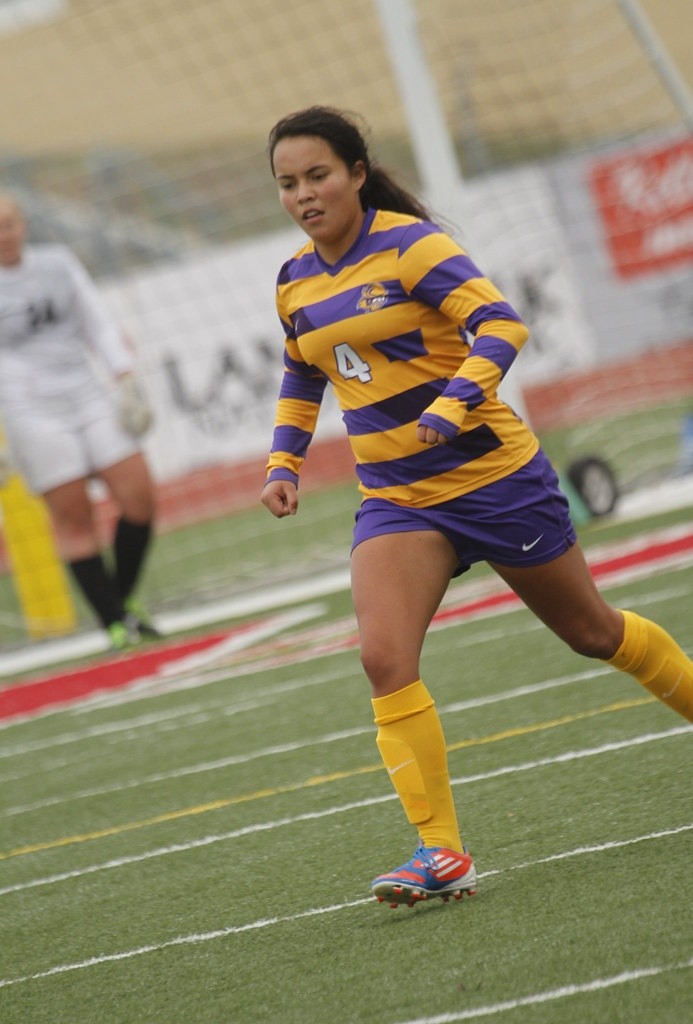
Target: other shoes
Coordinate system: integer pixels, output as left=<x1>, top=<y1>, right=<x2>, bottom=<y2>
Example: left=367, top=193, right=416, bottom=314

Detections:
left=124, top=594, right=163, bottom=639
left=107, top=620, right=129, bottom=658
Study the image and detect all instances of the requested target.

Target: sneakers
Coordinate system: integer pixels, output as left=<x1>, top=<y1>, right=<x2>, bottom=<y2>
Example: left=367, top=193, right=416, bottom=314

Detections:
left=371, top=837, right=476, bottom=909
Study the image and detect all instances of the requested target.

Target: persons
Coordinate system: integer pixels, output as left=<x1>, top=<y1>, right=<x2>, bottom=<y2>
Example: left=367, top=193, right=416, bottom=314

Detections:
left=259, top=103, right=693, bottom=910
left=0, top=191, right=166, bottom=654
